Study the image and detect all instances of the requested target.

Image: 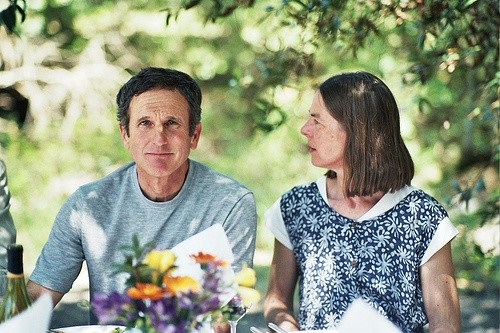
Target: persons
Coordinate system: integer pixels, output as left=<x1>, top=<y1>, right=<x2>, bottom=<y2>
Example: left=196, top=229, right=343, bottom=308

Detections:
left=25, top=67, right=258, bottom=333
left=263, top=71, right=461, bottom=333
left=0, top=160, right=17, bottom=298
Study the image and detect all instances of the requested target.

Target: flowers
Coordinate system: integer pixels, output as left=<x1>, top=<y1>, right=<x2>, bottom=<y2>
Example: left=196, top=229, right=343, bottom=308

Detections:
left=84, top=225, right=260, bottom=333
